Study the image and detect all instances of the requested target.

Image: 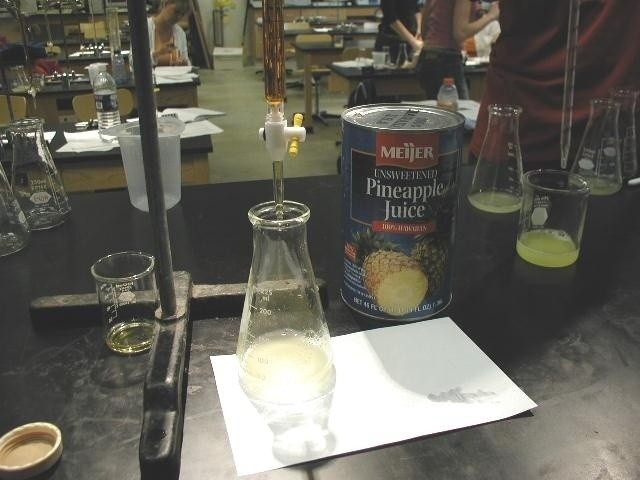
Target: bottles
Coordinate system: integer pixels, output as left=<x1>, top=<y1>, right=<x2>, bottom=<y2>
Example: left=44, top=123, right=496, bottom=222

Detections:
left=468, top=103, right=525, bottom=214
left=94, top=65, right=122, bottom=142
left=609, top=87, right=637, bottom=180
left=568, top=97, right=622, bottom=197
left=381, top=47, right=391, bottom=66
left=438, top=76, right=458, bottom=112
left=235, top=201, right=337, bottom=406
left=7, top=117, right=73, bottom=231
left=396, top=43, right=408, bottom=70
left=356, top=48, right=369, bottom=72
left=0, top=160, right=31, bottom=258
left=9, top=65, right=30, bottom=93
left=112, top=48, right=129, bottom=85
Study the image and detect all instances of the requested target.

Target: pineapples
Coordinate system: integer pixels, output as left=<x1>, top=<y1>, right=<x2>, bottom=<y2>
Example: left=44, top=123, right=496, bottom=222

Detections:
left=411, top=234, right=448, bottom=299
left=359, top=250, right=428, bottom=317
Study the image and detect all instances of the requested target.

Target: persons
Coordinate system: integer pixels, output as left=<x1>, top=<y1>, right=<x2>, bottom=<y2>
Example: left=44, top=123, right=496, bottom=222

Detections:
left=374, top=0, right=425, bottom=65
left=416, top=0, right=500, bottom=101
left=462, top=0, right=502, bottom=58
left=127, top=0, right=192, bottom=74
left=468, top=0, right=640, bottom=167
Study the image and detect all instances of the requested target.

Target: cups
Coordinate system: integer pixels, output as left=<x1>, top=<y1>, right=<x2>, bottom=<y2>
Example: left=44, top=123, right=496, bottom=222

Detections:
left=85, top=63, right=107, bottom=89
left=333, top=35, right=343, bottom=48
left=516, top=167, right=591, bottom=268
left=98, top=116, right=186, bottom=213
left=90, top=250, right=159, bottom=354
left=372, top=51, right=390, bottom=70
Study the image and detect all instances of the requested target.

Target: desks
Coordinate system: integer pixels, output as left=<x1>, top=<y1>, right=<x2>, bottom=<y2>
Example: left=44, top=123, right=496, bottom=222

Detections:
left=1, top=0, right=214, bottom=197
left=246, top=2, right=490, bottom=144
left=1, top=164, right=637, bottom=480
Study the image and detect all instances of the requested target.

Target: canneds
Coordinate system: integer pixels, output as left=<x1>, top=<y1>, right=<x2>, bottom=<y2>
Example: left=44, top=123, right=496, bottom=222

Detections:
left=341, top=103, right=466, bottom=322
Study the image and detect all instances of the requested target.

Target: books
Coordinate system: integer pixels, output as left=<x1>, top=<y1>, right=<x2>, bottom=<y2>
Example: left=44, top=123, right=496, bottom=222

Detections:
left=332, top=57, right=376, bottom=68
left=153, top=65, right=200, bottom=78
left=125, top=107, right=228, bottom=123
